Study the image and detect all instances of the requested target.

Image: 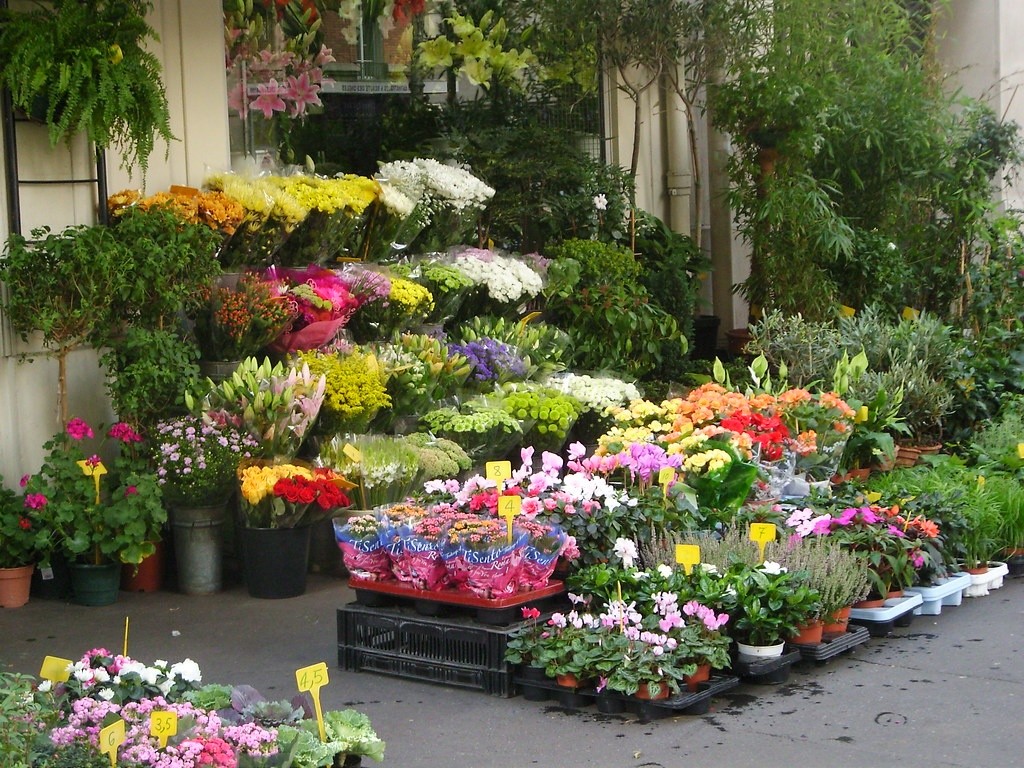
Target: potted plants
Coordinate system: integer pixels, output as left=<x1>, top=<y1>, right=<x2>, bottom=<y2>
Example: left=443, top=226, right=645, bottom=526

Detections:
left=716, top=59, right=808, bottom=361
left=659, top=0, right=774, bottom=361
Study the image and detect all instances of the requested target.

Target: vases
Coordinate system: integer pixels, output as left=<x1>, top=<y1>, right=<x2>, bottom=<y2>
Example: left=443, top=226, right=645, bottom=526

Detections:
left=1, top=89, right=1022, bottom=767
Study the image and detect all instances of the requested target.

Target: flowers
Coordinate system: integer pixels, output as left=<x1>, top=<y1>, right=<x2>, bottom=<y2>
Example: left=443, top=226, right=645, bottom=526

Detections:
left=1, top=157, right=1022, bottom=766
left=1, top=0, right=542, bottom=147
